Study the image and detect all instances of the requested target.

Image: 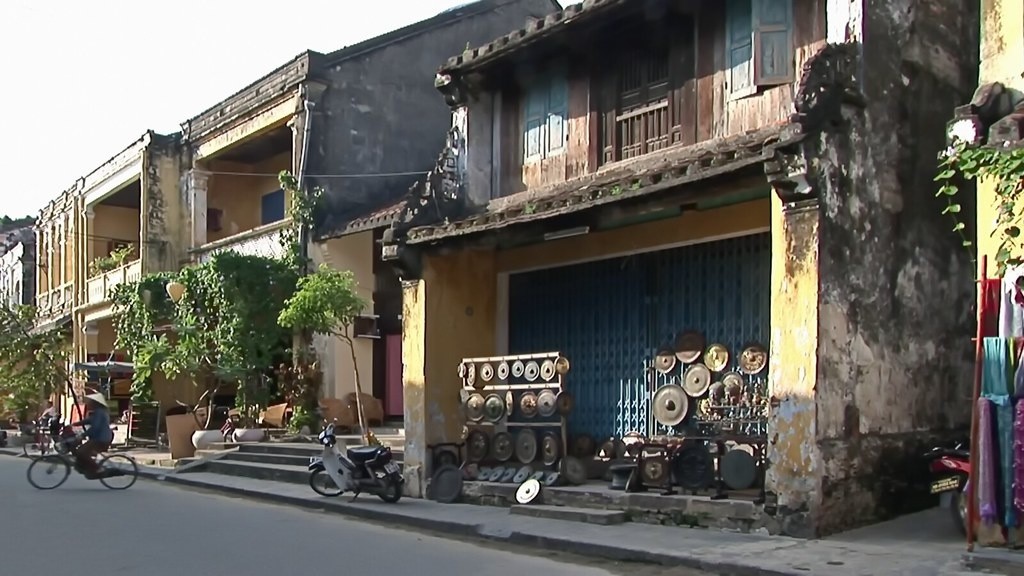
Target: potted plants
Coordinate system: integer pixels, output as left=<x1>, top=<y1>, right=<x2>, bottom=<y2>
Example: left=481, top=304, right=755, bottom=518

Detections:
left=108, top=251, right=302, bottom=450
left=3, top=385, right=44, bottom=432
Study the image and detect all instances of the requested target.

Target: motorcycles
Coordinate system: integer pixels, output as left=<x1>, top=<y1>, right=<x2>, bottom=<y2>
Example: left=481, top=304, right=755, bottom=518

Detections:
left=308, top=417, right=405, bottom=504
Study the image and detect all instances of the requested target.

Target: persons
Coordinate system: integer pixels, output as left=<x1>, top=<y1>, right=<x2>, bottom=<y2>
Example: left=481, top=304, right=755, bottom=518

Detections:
left=64, top=392, right=111, bottom=477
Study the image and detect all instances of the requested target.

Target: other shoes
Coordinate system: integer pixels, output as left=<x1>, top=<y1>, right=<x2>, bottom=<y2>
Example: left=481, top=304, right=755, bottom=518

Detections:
left=96, top=468, right=105, bottom=473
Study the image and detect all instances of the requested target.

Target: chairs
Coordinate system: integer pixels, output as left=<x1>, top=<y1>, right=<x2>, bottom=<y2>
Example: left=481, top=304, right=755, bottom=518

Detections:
left=348, top=391, right=384, bottom=428
left=319, top=397, right=354, bottom=433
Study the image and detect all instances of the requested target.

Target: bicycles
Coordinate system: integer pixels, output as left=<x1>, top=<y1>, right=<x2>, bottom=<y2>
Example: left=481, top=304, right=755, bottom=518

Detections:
left=26, top=424, right=140, bottom=490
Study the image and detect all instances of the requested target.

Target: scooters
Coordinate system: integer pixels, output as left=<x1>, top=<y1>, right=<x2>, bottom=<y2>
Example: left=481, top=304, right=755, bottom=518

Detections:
left=23, top=415, right=76, bottom=461
left=921, top=440, right=971, bottom=539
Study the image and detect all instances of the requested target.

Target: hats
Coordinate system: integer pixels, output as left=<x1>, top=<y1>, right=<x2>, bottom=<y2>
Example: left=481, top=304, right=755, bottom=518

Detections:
left=85, top=394, right=108, bottom=408
